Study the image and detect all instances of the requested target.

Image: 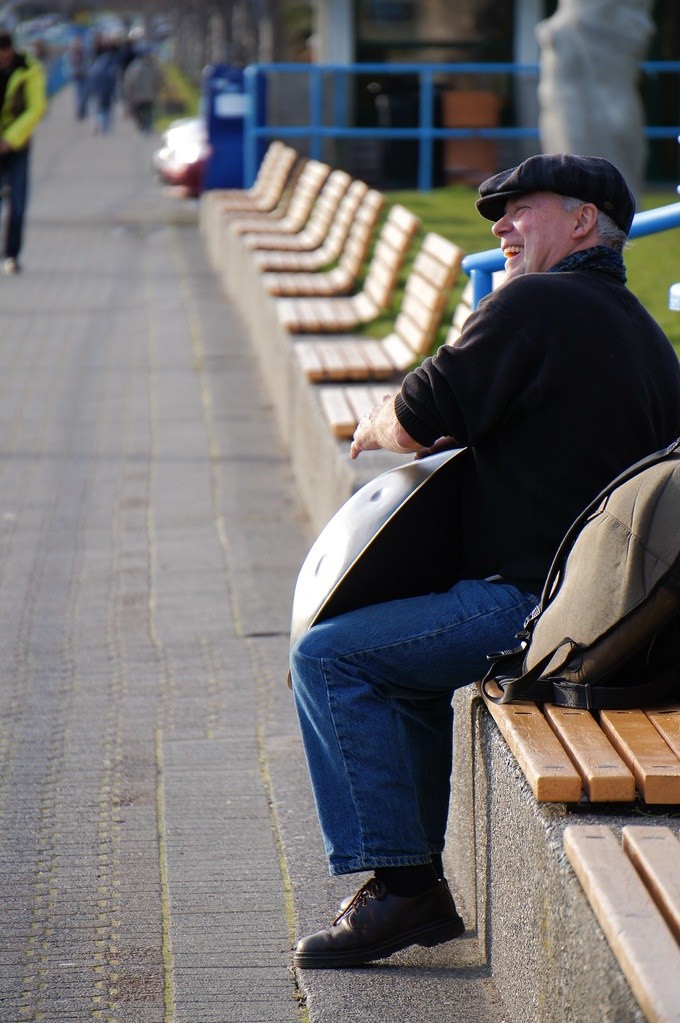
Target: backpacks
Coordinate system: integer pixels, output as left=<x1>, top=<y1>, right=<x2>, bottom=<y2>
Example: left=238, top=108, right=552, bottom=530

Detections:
left=479, top=437, right=679, bottom=709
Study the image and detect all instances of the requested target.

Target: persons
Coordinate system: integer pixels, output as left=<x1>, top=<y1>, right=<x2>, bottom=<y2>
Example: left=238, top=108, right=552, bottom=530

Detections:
left=66, top=25, right=167, bottom=136
left=292, top=157, right=680, bottom=970
left=0, top=18, right=46, bottom=276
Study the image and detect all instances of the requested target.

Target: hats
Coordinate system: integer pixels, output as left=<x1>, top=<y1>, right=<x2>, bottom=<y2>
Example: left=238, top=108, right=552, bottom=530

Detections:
left=475, top=153, right=636, bottom=237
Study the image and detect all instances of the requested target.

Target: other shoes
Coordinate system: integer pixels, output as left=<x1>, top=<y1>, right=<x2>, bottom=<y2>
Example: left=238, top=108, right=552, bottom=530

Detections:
left=6, top=258, right=18, bottom=272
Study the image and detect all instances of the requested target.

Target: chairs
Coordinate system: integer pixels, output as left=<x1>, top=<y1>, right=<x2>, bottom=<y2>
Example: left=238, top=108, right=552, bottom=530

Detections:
left=277, top=200, right=423, bottom=333
left=214, top=143, right=302, bottom=212
left=226, top=156, right=328, bottom=235
left=237, top=166, right=353, bottom=246
left=315, top=247, right=514, bottom=442
left=292, top=224, right=461, bottom=380
left=480, top=680, right=680, bottom=1023
left=198, top=130, right=285, bottom=200
left=255, top=175, right=370, bottom=274
left=262, top=187, right=391, bottom=296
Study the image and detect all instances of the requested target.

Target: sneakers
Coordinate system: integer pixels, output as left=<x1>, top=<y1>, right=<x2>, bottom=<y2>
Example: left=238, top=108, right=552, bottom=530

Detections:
left=293, top=876, right=465, bottom=968
